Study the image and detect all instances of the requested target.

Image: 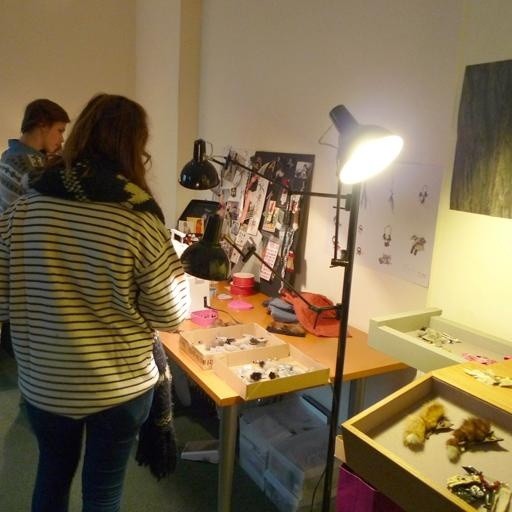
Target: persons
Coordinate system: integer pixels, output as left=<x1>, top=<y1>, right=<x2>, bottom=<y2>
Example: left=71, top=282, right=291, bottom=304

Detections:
left=0, top=92, right=193, bottom=511
left=219, top=153, right=311, bottom=235
left=280, top=200, right=298, bottom=261
left=0, top=98, right=72, bottom=220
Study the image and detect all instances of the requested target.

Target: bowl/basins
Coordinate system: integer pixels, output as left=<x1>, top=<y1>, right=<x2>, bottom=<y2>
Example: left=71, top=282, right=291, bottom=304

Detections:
left=233, top=273, right=255, bottom=288
left=230, top=282, right=256, bottom=297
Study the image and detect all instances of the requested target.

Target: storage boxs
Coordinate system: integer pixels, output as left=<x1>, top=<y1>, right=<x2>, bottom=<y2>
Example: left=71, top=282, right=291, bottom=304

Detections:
left=237, top=397, right=325, bottom=496
left=266, top=422, right=331, bottom=500
left=340, top=357, right=512, bottom=512
left=264, top=470, right=322, bottom=512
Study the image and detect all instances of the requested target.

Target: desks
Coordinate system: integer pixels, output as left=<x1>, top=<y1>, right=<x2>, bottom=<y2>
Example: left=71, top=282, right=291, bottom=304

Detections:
left=155, top=276, right=409, bottom=511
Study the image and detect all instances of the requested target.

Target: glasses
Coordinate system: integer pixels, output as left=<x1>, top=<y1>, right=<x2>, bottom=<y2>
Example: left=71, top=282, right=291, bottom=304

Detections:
left=140, top=150, right=151, bottom=165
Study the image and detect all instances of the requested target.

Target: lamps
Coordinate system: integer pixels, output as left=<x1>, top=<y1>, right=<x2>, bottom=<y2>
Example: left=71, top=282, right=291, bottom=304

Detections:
left=176, top=102, right=404, bottom=512
